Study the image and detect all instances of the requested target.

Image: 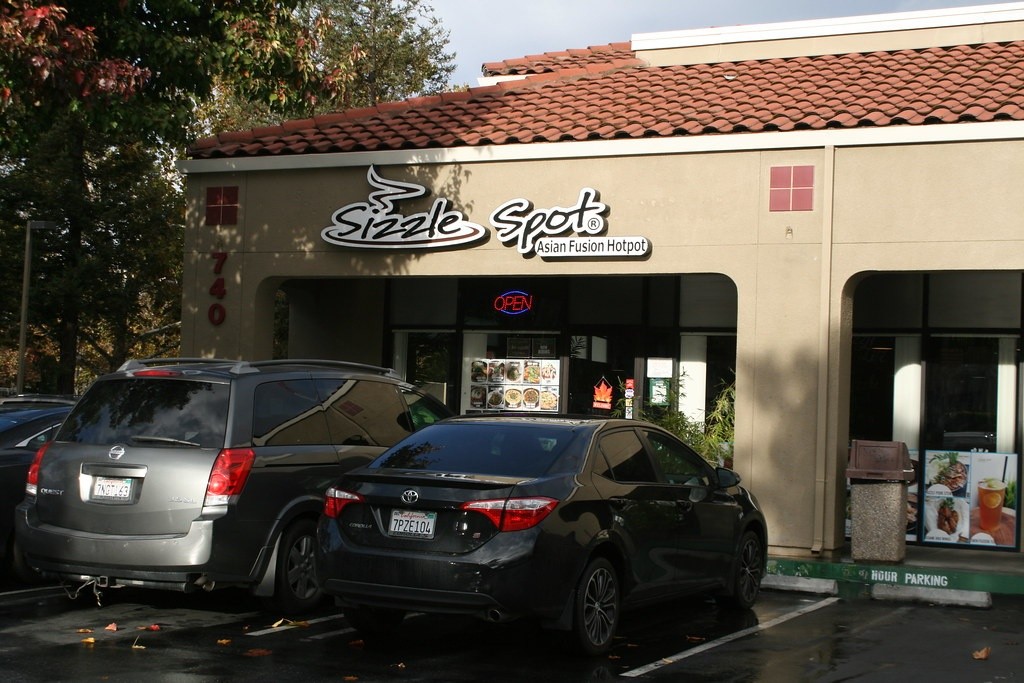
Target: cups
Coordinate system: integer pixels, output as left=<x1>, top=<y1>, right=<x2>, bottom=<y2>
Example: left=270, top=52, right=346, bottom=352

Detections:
left=976, top=479, right=1006, bottom=532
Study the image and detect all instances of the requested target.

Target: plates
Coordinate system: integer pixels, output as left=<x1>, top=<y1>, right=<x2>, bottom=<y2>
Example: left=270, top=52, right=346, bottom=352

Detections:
left=471, top=360, right=558, bottom=410
left=909, top=459, right=918, bottom=487
left=905, top=492, right=919, bottom=532
left=929, top=458, right=969, bottom=494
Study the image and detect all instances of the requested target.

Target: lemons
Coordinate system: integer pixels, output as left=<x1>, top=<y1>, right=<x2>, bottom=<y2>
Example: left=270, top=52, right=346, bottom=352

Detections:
left=984, top=493, right=1001, bottom=508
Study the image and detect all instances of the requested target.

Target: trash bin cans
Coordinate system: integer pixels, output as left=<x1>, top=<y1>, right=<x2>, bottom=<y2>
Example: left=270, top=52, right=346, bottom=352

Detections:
left=844, top=439, right=915, bottom=564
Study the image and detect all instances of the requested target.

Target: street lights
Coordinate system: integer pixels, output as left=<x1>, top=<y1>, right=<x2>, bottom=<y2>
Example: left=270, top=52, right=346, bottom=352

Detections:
left=15, top=221, right=56, bottom=396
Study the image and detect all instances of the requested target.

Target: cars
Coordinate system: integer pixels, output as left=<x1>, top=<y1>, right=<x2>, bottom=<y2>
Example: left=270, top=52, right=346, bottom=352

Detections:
left=0, top=408, right=76, bottom=586
left=313, top=417, right=770, bottom=659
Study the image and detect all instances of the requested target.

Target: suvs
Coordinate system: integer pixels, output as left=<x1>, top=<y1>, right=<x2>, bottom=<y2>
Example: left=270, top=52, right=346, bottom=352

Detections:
left=12, top=357, right=459, bottom=616
